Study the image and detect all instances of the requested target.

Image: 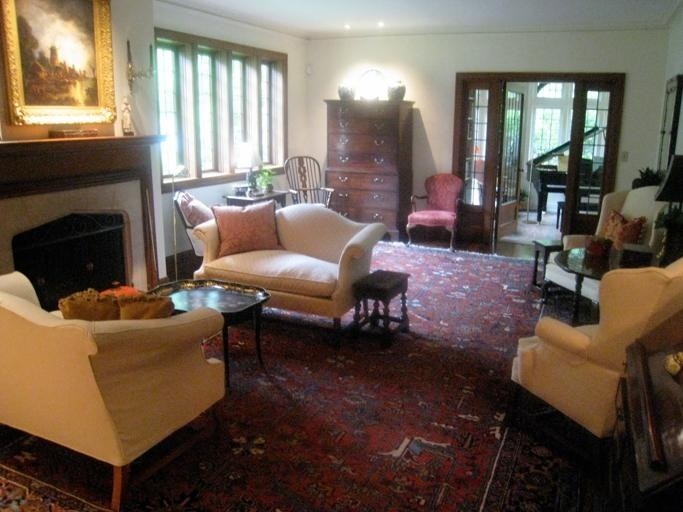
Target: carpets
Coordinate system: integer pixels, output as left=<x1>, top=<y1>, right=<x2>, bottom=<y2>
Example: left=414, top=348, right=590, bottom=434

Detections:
left=0, top=241, right=641, bottom=512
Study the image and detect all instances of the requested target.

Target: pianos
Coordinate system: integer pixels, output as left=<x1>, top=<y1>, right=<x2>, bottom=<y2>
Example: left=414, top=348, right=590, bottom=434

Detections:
left=526, top=126, right=602, bottom=224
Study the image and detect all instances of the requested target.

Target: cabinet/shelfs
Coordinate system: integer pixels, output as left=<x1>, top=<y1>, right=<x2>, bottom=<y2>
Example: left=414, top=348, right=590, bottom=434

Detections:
left=325, top=99, right=415, bottom=241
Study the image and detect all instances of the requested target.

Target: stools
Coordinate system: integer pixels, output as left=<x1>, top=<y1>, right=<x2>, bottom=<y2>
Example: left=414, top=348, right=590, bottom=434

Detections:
left=353, top=270, right=410, bottom=340
left=532, top=241, right=570, bottom=300
left=556, top=201, right=565, bottom=229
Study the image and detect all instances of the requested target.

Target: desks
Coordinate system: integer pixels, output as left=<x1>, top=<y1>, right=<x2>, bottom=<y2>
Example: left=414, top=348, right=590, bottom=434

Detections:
left=623, top=341, right=683, bottom=512
left=223, top=190, right=287, bottom=207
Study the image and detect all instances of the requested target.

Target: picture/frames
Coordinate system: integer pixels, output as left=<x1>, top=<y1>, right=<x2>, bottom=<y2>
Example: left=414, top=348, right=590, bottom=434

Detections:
left=0, top=0, right=115, bottom=125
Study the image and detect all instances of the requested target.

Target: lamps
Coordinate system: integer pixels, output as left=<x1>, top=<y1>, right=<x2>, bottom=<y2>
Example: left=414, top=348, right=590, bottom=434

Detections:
left=656, top=155, right=683, bottom=212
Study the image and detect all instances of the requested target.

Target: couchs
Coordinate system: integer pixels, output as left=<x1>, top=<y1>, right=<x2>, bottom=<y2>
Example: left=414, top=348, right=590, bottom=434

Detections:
left=0, top=271, right=227, bottom=512
left=174, top=191, right=214, bottom=256
left=193, top=205, right=386, bottom=331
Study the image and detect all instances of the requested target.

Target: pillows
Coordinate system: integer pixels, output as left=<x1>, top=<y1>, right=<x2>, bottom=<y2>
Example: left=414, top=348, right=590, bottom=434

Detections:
left=60, top=285, right=173, bottom=318
left=602, top=209, right=647, bottom=245
left=217, top=200, right=278, bottom=256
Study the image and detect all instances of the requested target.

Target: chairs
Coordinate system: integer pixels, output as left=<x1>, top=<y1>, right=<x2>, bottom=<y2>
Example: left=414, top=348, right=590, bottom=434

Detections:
left=284, top=154, right=333, bottom=205
left=511, top=257, right=682, bottom=437
left=542, top=185, right=679, bottom=305
left=405, top=174, right=464, bottom=250
